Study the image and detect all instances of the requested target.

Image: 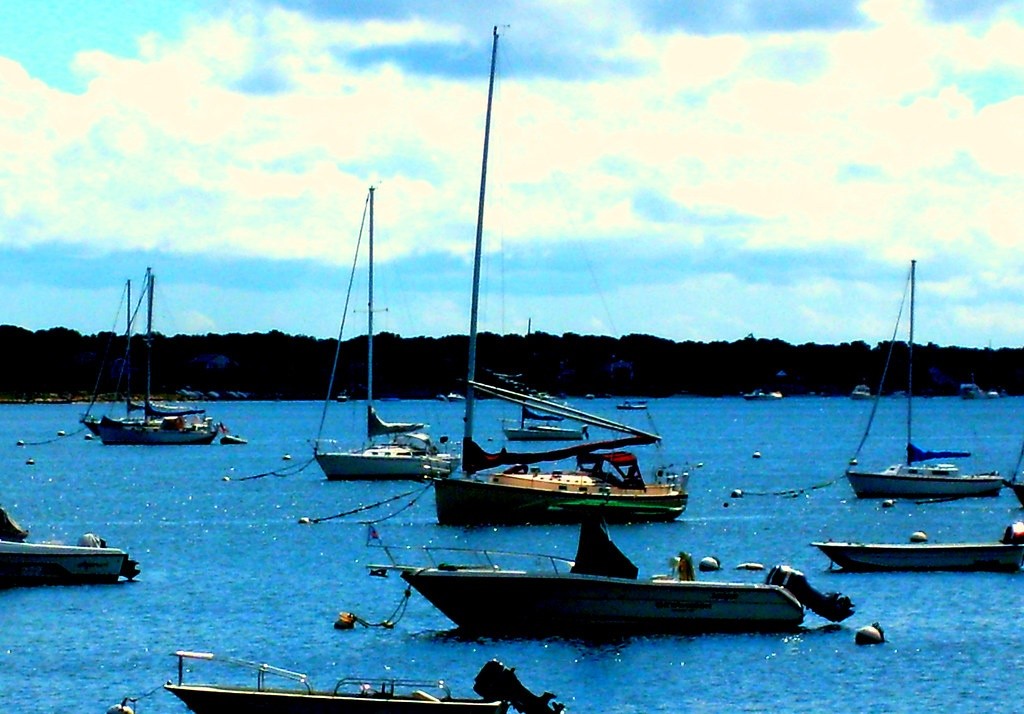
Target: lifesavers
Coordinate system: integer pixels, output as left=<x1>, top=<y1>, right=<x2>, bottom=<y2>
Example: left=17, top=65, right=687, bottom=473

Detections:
left=176, top=417, right=185, bottom=432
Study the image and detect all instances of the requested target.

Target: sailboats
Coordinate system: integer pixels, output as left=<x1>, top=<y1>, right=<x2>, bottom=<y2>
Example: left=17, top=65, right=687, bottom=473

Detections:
left=80, top=279, right=146, bottom=436
left=312, top=186, right=461, bottom=478
left=436, top=24, right=686, bottom=524
left=99, top=267, right=219, bottom=445
left=616, top=400, right=647, bottom=409
left=847, top=260, right=1003, bottom=499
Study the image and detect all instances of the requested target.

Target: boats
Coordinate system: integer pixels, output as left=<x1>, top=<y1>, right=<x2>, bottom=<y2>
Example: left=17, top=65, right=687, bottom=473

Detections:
left=811, top=521, right=1024, bottom=571
left=164, top=651, right=565, bottom=714
left=743, top=389, right=781, bottom=400
left=0, top=509, right=141, bottom=584
left=851, top=384, right=869, bottom=395
left=399, top=513, right=854, bottom=637
left=960, top=373, right=990, bottom=396
